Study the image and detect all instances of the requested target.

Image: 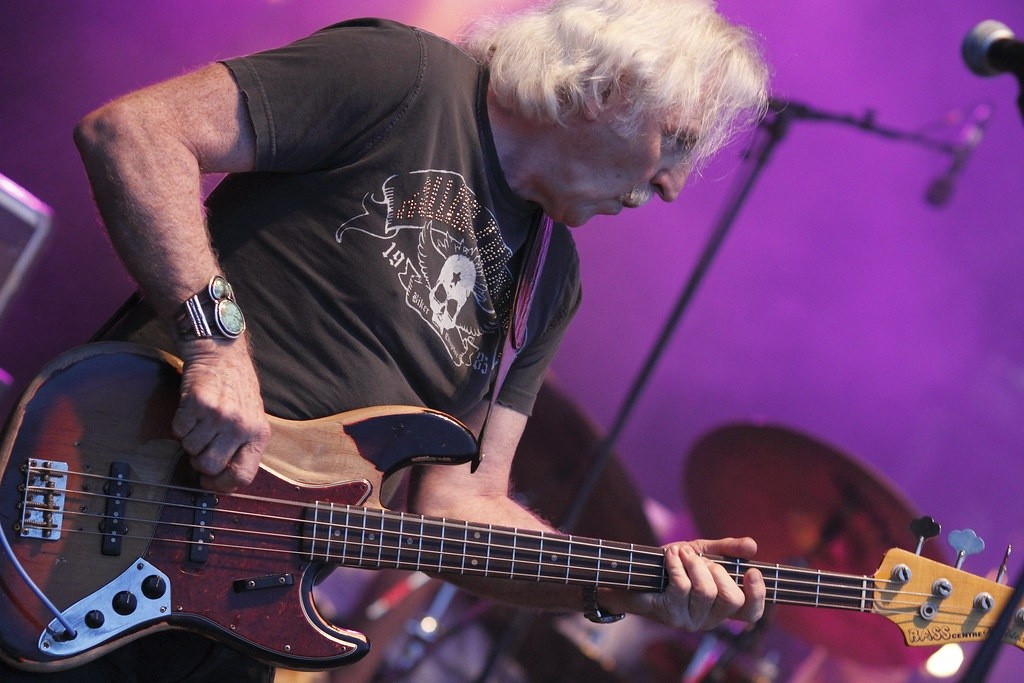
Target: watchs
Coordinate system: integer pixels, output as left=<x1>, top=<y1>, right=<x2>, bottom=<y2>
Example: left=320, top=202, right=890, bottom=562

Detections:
left=581, top=586, right=625, bottom=623
left=171, top=277, right=245, bottom=342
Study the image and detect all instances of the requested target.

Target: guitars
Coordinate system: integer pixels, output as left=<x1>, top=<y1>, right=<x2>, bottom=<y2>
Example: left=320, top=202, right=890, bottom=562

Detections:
left=0, top=338, right=1023, bottom=677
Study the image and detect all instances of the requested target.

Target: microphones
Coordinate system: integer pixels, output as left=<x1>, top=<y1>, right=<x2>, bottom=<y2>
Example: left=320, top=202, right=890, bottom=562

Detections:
left=962, top=19, right=1024, bottom=76
left=926, top=103, right=993, bottom=206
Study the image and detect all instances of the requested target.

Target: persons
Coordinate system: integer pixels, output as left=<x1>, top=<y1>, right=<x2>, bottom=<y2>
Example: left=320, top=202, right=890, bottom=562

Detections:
left=0, top=0, right=770, bottom=683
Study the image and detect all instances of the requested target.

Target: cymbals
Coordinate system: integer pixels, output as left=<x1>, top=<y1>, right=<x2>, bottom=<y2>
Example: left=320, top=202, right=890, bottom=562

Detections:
left=680, top=418, right=958, bottom=665
left=509, top=377, right=656, bottom=551
